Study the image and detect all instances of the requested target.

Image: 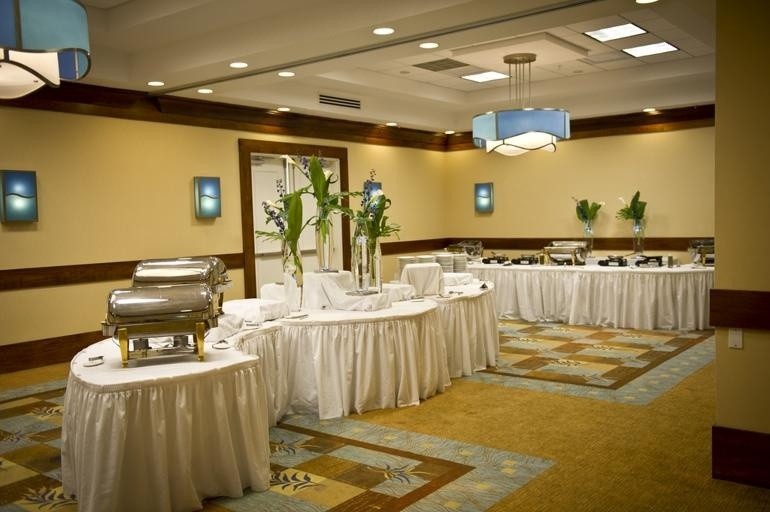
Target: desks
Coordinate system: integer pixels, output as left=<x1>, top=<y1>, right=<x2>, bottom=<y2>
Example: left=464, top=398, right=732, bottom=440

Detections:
left=69, top=271, right=499, bottom=511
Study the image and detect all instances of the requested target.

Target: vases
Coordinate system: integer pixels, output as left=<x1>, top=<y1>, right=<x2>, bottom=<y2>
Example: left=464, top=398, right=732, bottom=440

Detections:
left=634, top=226, right=644, bottom=254
left=314, top=208, right=334, bottom=270
left=281, top=255, right=305, bottom=312
left=352, top=237, right=382, bottom=295
left=584, top=226, right=594, bottom=254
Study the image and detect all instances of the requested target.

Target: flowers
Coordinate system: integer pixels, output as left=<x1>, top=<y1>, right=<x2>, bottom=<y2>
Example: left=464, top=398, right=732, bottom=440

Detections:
left=615, top=189, right=648, bottom=246
left=333, top=167, right=400, bottom=290
left=253, top=177, right=321, bottom=312
left=571, top=196, right=605, bottom=254
left=291, top=149, right=363, bottom=266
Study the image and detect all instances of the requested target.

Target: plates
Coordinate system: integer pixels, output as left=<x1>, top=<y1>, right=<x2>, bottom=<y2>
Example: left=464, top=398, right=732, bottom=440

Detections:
left=399, top=251, right=468, bottom=273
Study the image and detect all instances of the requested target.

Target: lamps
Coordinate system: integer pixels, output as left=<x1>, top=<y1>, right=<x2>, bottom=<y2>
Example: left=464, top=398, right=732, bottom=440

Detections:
left=471, top=52, right=571, bottom=158
left=0, top=0, right=92, bottom=99
